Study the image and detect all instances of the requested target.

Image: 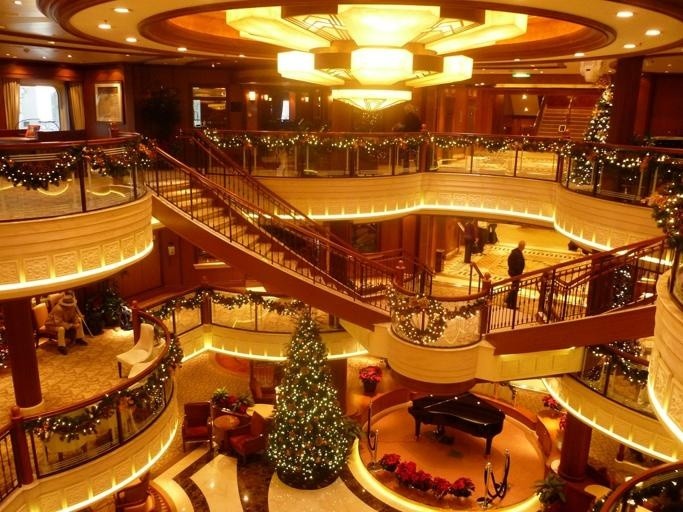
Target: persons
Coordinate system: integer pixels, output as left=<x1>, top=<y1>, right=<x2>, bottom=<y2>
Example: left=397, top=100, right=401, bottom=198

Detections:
left=394, top=102, right=421, bottom=176
left=503, top=239, right=526, bottom=312
left=43, top=294, right=87, bottom=357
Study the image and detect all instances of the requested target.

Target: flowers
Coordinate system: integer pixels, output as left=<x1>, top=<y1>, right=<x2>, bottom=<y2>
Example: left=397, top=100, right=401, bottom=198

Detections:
left=379, top=453, right=475, bottom=497
left=358, top=365, right=383, bottom=384
left=211, top=387, right=255, bottom=412
left=541, top=395, right=562, bottom=412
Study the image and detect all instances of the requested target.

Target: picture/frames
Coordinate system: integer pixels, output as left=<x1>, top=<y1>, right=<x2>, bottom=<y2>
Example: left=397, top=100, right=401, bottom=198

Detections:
left=349, top=220, right=383, bottom=254
left=92, top=80, right=127, bottom=125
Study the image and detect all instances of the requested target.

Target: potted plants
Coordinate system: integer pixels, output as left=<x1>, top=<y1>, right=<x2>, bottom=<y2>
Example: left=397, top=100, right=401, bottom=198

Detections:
left=533, top=470, right=568, bottom=512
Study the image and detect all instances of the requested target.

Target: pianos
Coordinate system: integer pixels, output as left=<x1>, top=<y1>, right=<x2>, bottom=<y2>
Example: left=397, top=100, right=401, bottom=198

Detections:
left=409, top=391, right=505, bottom=454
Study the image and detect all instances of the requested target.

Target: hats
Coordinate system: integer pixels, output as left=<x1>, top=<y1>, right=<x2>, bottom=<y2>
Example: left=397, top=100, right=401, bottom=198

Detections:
left=58, top=295, right=77, bottom=307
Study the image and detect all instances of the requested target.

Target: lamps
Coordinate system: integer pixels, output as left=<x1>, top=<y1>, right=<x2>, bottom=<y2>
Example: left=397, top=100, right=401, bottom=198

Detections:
left=225, top=0, right=530, bottom=112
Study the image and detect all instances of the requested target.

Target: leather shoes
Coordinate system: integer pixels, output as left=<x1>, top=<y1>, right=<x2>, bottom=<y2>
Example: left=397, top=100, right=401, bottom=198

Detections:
left=398, top=171, right=409, bottom=175
left=56, top=338, right=88, bottom=355
left=506, top=304, right=519, bottom=310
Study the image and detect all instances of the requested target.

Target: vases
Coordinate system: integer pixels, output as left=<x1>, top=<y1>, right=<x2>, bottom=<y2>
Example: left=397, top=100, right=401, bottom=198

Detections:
left=361, top=382, right=378, bottom=397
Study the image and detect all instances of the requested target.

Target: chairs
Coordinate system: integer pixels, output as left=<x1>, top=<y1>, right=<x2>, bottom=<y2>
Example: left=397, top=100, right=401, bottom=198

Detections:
left=115, top=322, right=166, bottom=392
left=182, top=402, right=267, bottom=464
left=28, top=291, right=86, bottom=353
